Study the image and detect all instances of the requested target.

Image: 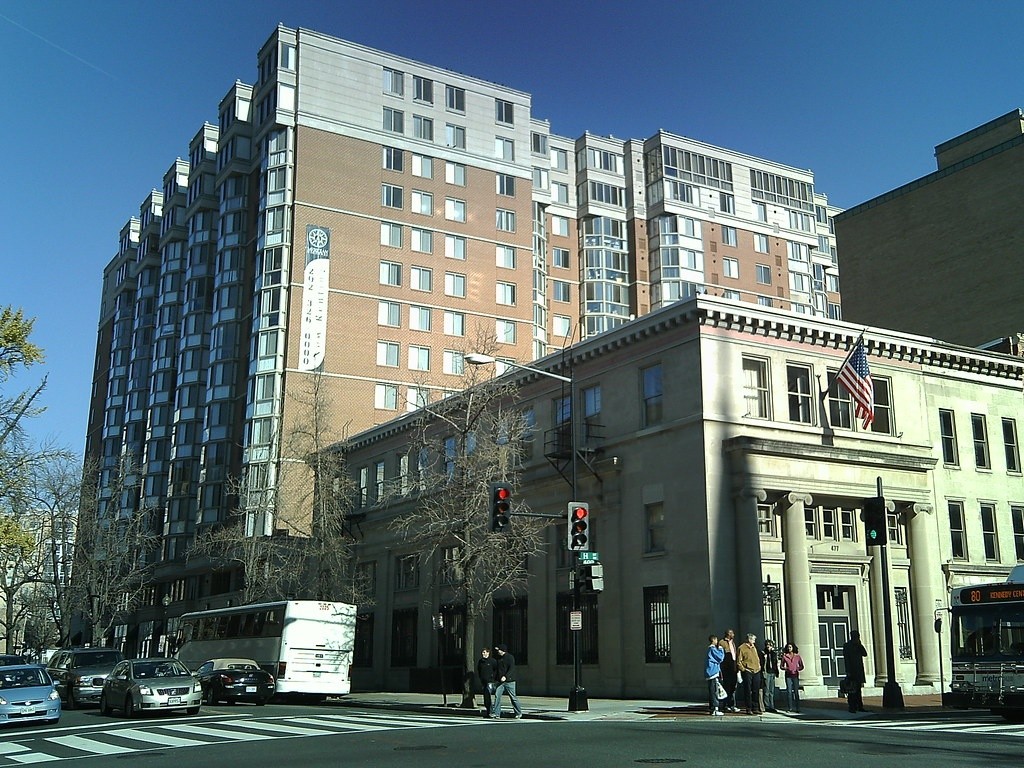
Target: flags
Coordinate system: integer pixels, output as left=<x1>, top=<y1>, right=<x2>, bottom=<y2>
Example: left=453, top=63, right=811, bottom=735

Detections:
left=834, top=334, right=874, bottom=430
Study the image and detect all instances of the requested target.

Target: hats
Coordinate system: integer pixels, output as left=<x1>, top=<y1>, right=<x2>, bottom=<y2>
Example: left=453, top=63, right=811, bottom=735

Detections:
left=495, top=644, right=507, bottom=652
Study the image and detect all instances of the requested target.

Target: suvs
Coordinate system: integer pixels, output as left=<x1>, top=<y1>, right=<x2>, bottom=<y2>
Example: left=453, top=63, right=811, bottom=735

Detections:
left=45, top=644, right=124, bottom=711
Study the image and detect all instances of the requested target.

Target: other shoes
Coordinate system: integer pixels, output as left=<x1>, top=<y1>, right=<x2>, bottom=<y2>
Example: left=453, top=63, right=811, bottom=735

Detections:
left=858, top=708, right=868, bottom=712
left=515, top=713, right=523, bottom=719
left=489, top=713, right=500, bottom=719
left=849, top=709, right=856, bottom=713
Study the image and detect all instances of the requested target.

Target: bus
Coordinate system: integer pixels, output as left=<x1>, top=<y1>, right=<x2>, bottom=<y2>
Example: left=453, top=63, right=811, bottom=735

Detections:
left=933, top=566, right=1024, bottom=724
left=164, top=598, right=357, bottom=704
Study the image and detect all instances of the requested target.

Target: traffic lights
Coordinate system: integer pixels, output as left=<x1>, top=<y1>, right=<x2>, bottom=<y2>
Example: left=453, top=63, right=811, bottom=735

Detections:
left=573, top=506, right=588, bottom=551
left=494, top=487, right=511, bottom=533
left=578, top=564, right=604, bottom=593
left=864, top=498, right=890, bottom=550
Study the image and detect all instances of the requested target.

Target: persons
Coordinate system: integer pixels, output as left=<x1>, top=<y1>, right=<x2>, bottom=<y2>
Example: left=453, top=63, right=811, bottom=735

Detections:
left=153, top=663, right=174, bottom=677
left=0, top=670, right=35, bottom=689
left=843, top=630, right=868, bottom=714
left=780, top=643, right=804, bottom=713
left=704, top=635, right=725, bottom=716
left=717, top=628, right=740, bottom=713
left=478, top=643, right=522, bottom=719
left=759, top=639, right=777, bottom=713
left=736, top=633, right=763, bottom=715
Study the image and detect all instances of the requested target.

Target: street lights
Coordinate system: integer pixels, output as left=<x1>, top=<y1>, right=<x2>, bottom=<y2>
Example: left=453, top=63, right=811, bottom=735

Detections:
left=465, top=351, right=590, bottom=713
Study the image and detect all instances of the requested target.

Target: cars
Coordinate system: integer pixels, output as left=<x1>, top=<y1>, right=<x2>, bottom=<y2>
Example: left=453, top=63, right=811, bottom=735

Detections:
left=191, top=658, right=276, bottom=706
left=92, top=657, right=203, bottom=721
left=0, top=653, right=62, bottom=729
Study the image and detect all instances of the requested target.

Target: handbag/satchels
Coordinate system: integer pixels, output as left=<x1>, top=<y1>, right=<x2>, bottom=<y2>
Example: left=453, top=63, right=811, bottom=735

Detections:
left=715, top=678, right=728, bottom=700
left=840, top=674, right=859, bottom=693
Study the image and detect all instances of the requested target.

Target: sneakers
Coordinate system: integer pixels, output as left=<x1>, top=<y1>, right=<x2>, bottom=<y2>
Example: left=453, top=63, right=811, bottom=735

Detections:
left=747, top=710, right=762, bottom=715
left=725, top=706, right=740, bottom=713
left=712, top=710, right=725, bottom=716
